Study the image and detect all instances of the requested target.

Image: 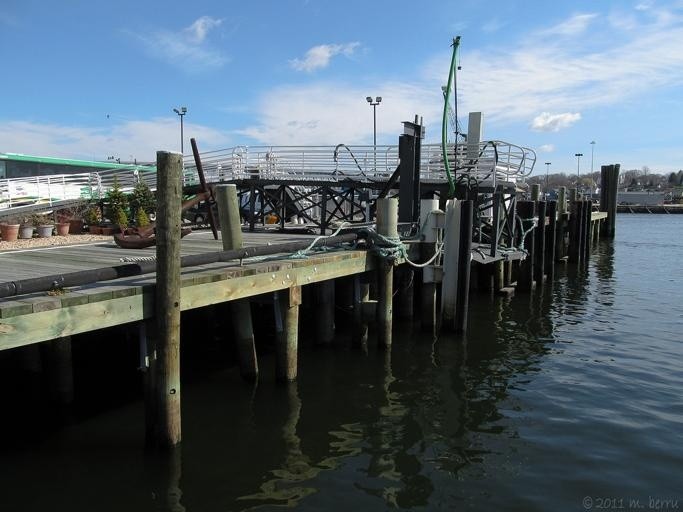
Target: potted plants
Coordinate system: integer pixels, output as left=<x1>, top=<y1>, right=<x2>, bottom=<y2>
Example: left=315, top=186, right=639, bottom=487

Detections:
left=33, top=203, right=113, bottom=238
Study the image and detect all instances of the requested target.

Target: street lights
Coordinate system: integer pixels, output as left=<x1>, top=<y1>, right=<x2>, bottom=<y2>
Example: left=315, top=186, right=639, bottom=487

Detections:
left=590, top=141, right=596, bottom=172
left=545, top=162, right=551, bottom=187
left=366, top=96, right=382, bottom=176
left=173, top=106, right=187, bottom=153
left=574, top=153, right=584, bottom=177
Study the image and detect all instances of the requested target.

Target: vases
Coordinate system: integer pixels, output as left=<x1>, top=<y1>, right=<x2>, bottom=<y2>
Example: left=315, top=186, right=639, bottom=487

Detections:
left=1, top=224, right=32, bottom=241
